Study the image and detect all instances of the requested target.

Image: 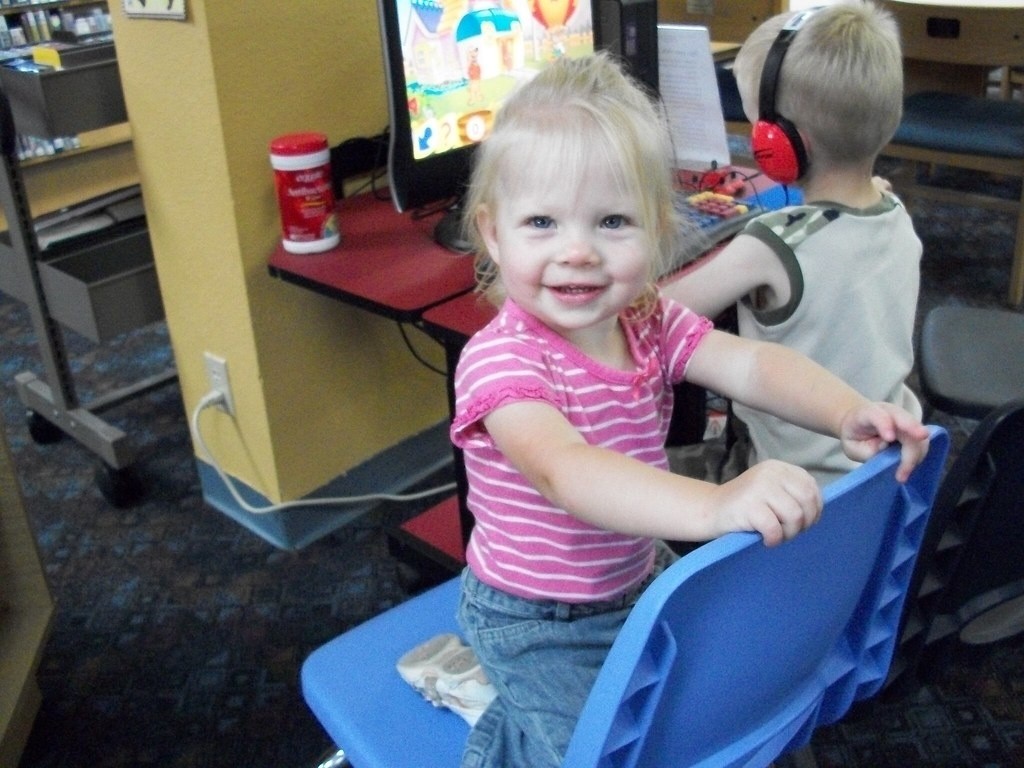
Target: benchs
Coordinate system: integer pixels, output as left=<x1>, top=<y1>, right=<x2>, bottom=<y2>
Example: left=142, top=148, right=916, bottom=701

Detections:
left=721, top=64, right=1023, bottom=311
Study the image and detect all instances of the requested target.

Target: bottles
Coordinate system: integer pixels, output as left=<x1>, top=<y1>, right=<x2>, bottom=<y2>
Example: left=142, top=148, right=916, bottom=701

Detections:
left=269, top=131, right=340, bottom=254
left=75, top=18, right=90, bottom=35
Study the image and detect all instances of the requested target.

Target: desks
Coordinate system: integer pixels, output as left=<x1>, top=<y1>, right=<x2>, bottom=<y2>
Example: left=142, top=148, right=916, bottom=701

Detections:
left=270, top=165, right=812, bottom=569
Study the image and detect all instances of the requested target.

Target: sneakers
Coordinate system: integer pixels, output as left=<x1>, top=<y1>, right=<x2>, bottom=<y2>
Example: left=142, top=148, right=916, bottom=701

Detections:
left=395, top=633, right=499, bottom=709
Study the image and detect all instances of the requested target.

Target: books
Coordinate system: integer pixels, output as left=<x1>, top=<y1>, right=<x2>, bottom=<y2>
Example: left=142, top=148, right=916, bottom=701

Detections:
left=14, top=134, right=80, bottom=160
left=0, top=10, right=51, bottom=48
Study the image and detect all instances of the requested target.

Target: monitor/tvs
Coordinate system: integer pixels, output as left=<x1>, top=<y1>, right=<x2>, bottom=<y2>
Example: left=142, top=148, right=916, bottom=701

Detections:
left=376, top=0, right=602, bottom=254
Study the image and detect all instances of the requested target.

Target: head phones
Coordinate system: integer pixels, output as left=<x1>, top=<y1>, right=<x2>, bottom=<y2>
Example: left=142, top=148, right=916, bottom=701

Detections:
left=749, top=5, right=826, bottom=184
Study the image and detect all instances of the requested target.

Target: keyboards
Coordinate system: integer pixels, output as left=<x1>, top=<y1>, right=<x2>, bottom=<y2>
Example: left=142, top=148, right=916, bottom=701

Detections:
left=653, top=188, right=769, bottom=278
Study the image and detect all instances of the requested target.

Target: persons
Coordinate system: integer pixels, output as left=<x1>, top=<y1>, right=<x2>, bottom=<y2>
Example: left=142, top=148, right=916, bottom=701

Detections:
left=450, top=55, right=929, bottom=768
left=660, top=0, right=923, bottom=490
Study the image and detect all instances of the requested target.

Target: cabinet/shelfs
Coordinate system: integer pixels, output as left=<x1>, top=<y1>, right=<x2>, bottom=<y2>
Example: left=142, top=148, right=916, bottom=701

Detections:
left=2, top=4, right=174, bottom=505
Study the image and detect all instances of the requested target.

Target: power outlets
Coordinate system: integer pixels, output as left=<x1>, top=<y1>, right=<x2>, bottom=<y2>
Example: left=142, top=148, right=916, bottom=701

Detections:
left=200, top=354, right=233, bottom=417
left=122, top=0, right=188, bottom=19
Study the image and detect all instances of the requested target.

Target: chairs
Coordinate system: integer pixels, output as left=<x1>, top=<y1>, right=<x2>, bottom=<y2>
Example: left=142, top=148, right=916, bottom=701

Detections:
left=298, top=427, right=949, bottom=768
left=898, top=399, right=1023, bottom=668
left=889, top=64, right=1024, bottom=308
left=918, top=303, right=1024, bottom=420
